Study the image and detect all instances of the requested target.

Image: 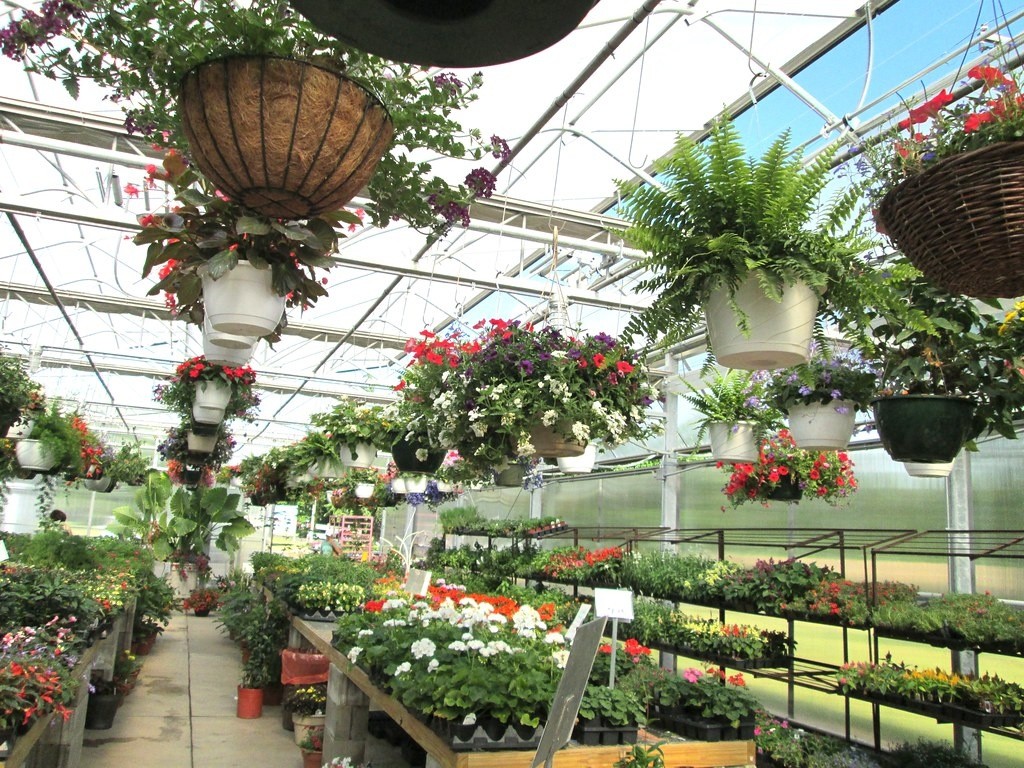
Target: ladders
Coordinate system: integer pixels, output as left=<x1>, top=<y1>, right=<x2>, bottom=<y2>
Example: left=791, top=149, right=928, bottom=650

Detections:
left=340, top=516, right=373, bottom=562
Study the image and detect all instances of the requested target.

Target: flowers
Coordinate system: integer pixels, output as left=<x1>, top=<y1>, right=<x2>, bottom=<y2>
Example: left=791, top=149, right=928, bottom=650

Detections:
left=741, top=341, right=887, bottom=415
left=0, top=503, right=1023, bottom=766
left=1, top=2, right=512, bottom=239
left=716, top=428, right=858, bottom=510
left=1, top=244, right=651, bottom=530
left=856, top=45, right=1023, bottom=231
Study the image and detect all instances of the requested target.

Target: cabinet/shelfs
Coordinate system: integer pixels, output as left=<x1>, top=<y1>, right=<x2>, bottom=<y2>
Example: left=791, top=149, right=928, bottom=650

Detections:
left=443, top=528, right=577, bottom=598
left=871, top=529, right=1023, bottom=768
left=508, top=527, right=670, bottom=671
left=616, top=529, right=918, bottom=768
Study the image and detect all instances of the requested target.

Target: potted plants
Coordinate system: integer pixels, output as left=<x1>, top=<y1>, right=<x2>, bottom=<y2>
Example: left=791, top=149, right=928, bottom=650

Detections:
left=867, top=268, right=1023, bottom=463
left=611, top=117, right=941, bottom=370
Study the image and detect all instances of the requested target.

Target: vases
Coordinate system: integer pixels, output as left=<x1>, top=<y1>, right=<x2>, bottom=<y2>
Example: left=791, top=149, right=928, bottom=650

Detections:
left=390, top=433, right=447, bottom=475
left=325, top=490, right=332, bottom=504
left=5, top=415, right=34, bottom=439
left=169, top=564, right=196, bottom=606
left=178, top=471, right=200, bottom=485
left=291, top=713, right=325, bottom=746
left=250, top=494, right=268, bottom=507
left=14, top=439, right=56, bottom=471
left=314, top=459, right=343, bottom=479
left=201, top=321, right=251, bottom=366
left=113, top=676, right=130, bottom=696
left=181, top=446, right=204, bottom=464
left=509, top=412, right=589, bottom=458
left=203, top=305, right=257, bottom=349
left=435, top=480, right=455, bottom=493
left=495, top=464, right=523, bottom=487
left=194, top=608, right=209, bottom=616
left=391, top=476, right=427, bottom=493
left=84, top=688, right=122, bottom=730
left=229, top=627, right=234, bottom=640
left=339, top=439, right=377, bottom=468
left=181, top=57, right=393, bottom=217
left=127, top=475, right=143, bottom=486
left=469, top=481, right=483, bottom=490
left=192, top=380, right=232, bottom=410
left=301, top=748, right=322, bottom=768
left=84, top=476, right=110, bottom=491
left=189, top=398, right=225, bottom=424
left=879, top=141, right=1023, bottom=298
left=187, top=408, right=220, bottom=436
left=903, top=458, right=955, bottom=477
left=84, top=465, right=102, bottom=480
left=765, top=472, right=803, bottom=500
left=96, top=479, right=116, bottom=493
left=195, top=260, right=285, bottom=336
left=118, top=670, right=137, bottom=689
left=354, top=483, right=373, bottom=498
left=0, top=414, right=13, bottom=439
left=183, top=428, right=218, bottom=452
left=785, top=398, right=860, bottom=450
left=287, top=471, right=309, bottom=489
